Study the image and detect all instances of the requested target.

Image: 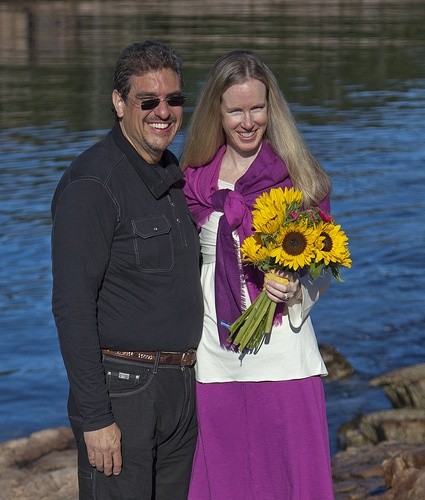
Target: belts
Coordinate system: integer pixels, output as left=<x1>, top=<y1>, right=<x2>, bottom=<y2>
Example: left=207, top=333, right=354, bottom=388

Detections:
left=101, top=349, right=196, bottom=366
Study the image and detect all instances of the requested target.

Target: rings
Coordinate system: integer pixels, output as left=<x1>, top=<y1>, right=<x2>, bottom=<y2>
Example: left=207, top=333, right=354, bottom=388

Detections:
left=284, top=294, right=290, bottom=301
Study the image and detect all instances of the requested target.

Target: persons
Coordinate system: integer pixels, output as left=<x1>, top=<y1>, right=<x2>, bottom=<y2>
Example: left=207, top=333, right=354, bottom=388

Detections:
left=178, top=51, right=331, bottom=500
left=50, top=41, right=203, bottom=500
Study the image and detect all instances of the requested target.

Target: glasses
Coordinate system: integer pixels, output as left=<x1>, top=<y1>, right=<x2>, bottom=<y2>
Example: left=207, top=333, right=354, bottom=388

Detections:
left=126, top=95, right=186, bottom=111
